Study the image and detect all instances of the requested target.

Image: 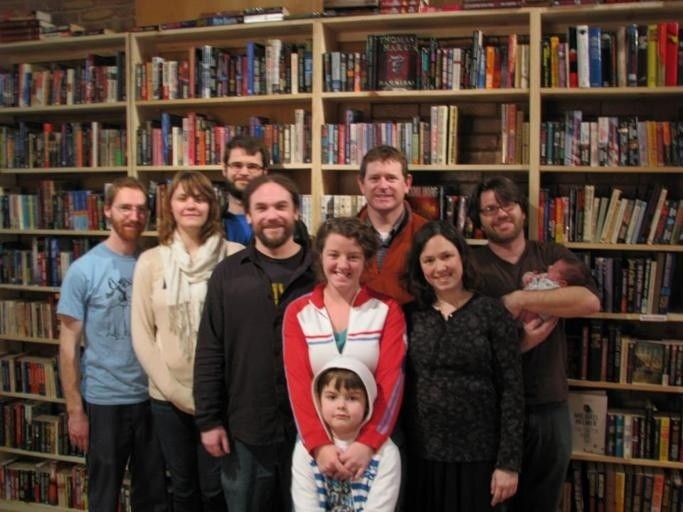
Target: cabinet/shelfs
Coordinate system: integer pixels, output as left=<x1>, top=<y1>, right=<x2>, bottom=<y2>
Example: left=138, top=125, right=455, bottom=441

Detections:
left=316, top=11, right=535, bottom=244
left=2, top=32, right=134, bottom=512
left=536, top=1, right=682, bottom=510
left=132, top=20, right=316, bottom=237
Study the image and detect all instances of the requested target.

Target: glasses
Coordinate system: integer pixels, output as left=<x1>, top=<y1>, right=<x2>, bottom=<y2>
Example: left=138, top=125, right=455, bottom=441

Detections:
left=477, top=200, right=518, bottom=217
left=225, top=162, right=265, bottom=174
